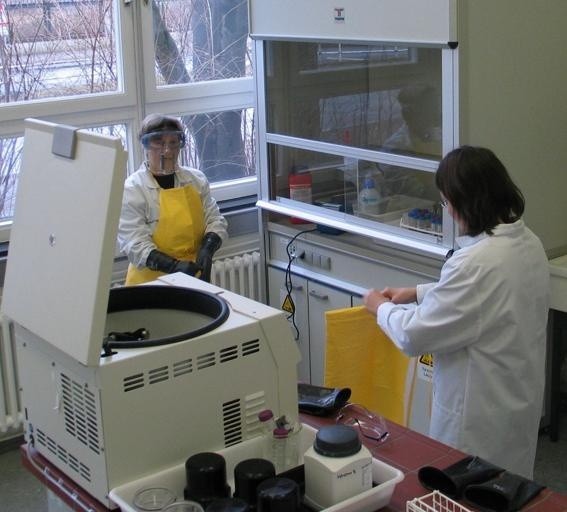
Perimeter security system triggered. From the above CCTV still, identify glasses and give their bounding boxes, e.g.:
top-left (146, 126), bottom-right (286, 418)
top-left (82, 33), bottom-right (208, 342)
top-left (440, 199), bottom-right (450, 207)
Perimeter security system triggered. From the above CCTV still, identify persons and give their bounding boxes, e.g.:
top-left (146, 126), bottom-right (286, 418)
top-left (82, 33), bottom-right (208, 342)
top-left (116, 114), bottom-right (228, 286)
top-left (366, 83), bottom-right (443, 226)
top-left (365, 145), bottom-right (549, 482)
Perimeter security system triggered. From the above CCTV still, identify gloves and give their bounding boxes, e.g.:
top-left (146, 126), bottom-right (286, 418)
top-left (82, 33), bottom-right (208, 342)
top-left (298, 383), bottom-right (351, 416)
top-left (417, 455), bottom-right (546, 510)
top-left (146, 231), bottom-right (222, 282)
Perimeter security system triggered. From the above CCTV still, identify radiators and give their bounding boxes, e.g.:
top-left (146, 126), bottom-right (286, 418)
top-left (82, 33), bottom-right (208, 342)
top-left (212, 251), bottom-right (263, 303)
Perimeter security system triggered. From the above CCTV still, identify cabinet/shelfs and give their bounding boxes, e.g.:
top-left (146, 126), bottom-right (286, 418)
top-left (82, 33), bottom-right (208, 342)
top-left (265, 265), bottom-right (352, 386)
top-left (253, 34), bottom-right (567, 258)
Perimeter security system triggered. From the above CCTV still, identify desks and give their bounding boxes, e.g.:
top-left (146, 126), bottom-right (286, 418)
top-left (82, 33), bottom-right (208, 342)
top-left (19, 380), bottom-right (567, 512)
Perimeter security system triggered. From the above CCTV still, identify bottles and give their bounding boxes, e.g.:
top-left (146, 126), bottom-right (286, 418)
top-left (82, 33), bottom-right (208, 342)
top-left (185, 409), bottom-right (372, 512)
top-left (289, 166), bottom-right (314, 225)
top-left (133, 486), bottom-right (205, 511)
top-left (402, 208), bottom-right (442, 235)
top-left (358, 178), bottom-right (380, 217)
